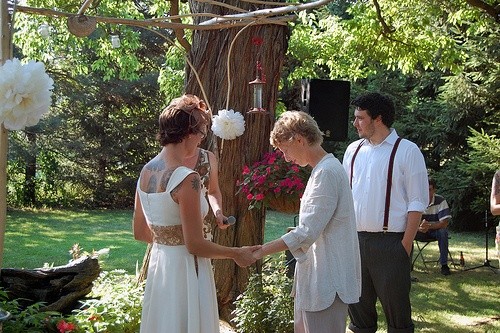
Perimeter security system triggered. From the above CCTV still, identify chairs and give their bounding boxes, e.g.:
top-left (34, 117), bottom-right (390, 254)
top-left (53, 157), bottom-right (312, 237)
top-left (411, 234), bottom-right (457, 272)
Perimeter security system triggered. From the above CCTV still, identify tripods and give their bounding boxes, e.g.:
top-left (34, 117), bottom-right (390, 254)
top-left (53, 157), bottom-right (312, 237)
top-left (462, 186), bottom-right (498, 271)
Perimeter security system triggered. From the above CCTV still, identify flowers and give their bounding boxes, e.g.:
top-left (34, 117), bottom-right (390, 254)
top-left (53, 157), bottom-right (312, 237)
top-left (210, 109), bottom-right (245, 141)
top-left (234, 149), bottom-right (313, 212)
top-left (0, 57), bottom-right (54, 130)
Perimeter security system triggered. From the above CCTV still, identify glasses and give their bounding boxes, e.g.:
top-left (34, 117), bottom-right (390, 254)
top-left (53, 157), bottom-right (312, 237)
top-left (197, 129), bottom-right (208, 139)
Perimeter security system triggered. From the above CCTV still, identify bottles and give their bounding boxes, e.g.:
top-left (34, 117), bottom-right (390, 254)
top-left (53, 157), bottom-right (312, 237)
top-left (460, 251), bottom-right (464, 268)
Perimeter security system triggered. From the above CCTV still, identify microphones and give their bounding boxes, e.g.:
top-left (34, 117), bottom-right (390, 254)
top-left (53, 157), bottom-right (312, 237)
top-left (223, 216), bottom-right (236, 226)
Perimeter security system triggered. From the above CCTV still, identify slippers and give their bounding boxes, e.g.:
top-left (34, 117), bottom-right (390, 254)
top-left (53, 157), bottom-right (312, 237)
top-left (441, 265), bottom-right (450, 276)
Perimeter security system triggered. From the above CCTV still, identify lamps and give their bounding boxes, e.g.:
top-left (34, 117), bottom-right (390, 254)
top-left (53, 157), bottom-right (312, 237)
top-left (246, 61), bottom-right (266, 113)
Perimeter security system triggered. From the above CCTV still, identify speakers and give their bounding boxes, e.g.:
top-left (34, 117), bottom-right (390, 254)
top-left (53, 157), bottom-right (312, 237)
top-left (308, 78), bottom-right (350, 141)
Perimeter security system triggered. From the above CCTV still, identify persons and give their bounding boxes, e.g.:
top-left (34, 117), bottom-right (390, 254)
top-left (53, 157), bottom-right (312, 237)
top-left (410, 179), bottom-right (451, 275)
top-left (133, 95), bottom-right (262, 333)
top-left (343, 93), bottom-right (430, 333)
top-left (490, 170), bottom-right (500, 259)
top-left (184, 148), bottom-right (230, 229)
top-left (253, 111), bottom-right (362, 333)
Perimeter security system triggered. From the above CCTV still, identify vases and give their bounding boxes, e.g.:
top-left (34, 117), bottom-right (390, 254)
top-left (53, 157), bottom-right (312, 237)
top-left (267, 195), bottom-right (299, 214)
